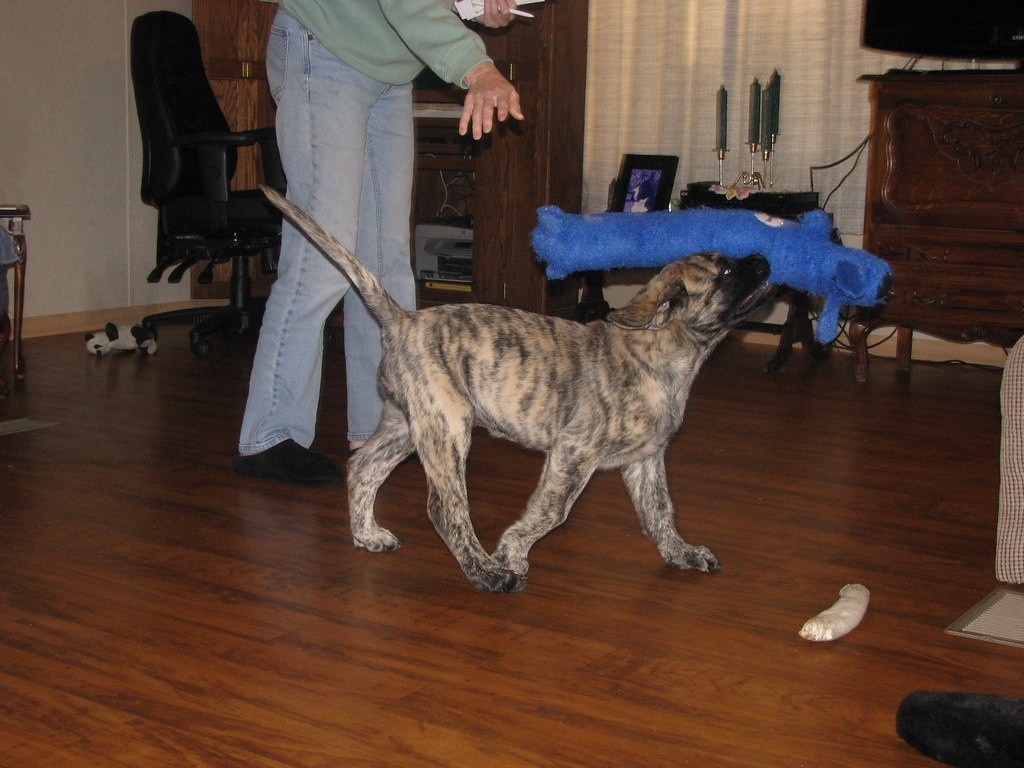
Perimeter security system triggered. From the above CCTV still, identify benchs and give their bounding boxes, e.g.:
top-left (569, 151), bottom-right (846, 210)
top-left (564, 191), bottom-right (830, 374)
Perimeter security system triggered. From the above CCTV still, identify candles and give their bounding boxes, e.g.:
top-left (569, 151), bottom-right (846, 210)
top-left (748, 76), bottom-right (761, 142)
top-left (759, 67), bottom-right (780, 149)
top-left (714, 84), bottom-right (729, 149)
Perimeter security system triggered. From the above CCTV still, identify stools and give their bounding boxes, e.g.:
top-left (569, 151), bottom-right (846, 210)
top-left (0, 204), bottom-right (31, 397)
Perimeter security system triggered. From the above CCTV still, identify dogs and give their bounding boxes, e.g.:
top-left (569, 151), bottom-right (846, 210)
top-left (251, 182), bottom-right (774, 592)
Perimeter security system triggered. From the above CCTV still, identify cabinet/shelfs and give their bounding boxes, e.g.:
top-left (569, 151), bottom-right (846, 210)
top-left (849, 68), bottom-right (1024, 383)
top-left (189, 0), bottom-right (587, 313)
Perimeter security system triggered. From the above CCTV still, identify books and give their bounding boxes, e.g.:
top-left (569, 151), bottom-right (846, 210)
top-left (413, 102), bottom-right (463, 118)
top-left (425, 282), bottom-right (472, 293)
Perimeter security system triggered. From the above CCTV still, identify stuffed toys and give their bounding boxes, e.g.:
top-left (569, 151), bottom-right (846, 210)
top-left (85, 323), bottom-right (157, 356)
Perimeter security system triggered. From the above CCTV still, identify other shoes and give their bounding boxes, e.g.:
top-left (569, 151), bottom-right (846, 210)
top-left (896, 688), bottom-right (1024, 768)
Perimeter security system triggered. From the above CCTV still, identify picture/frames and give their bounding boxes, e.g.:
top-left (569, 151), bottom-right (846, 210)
top-left (610, 153), bottom-right (680, 212)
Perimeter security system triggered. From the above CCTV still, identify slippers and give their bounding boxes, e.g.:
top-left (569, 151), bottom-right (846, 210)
top-left (236, 437), bottom-right (335, 486)
top-left (346, 445), bottom-right (419, 464)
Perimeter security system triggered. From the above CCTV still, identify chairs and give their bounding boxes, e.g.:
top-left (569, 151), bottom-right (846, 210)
top-left (131, 11), bottom-right (336, 358)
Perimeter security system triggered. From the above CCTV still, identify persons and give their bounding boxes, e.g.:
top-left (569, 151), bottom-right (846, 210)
top-left (241, 0), bottom-right (526, 487)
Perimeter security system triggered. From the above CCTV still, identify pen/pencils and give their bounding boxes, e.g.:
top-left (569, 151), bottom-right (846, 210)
top-left (471, 0), bottom-right (535, 18)
